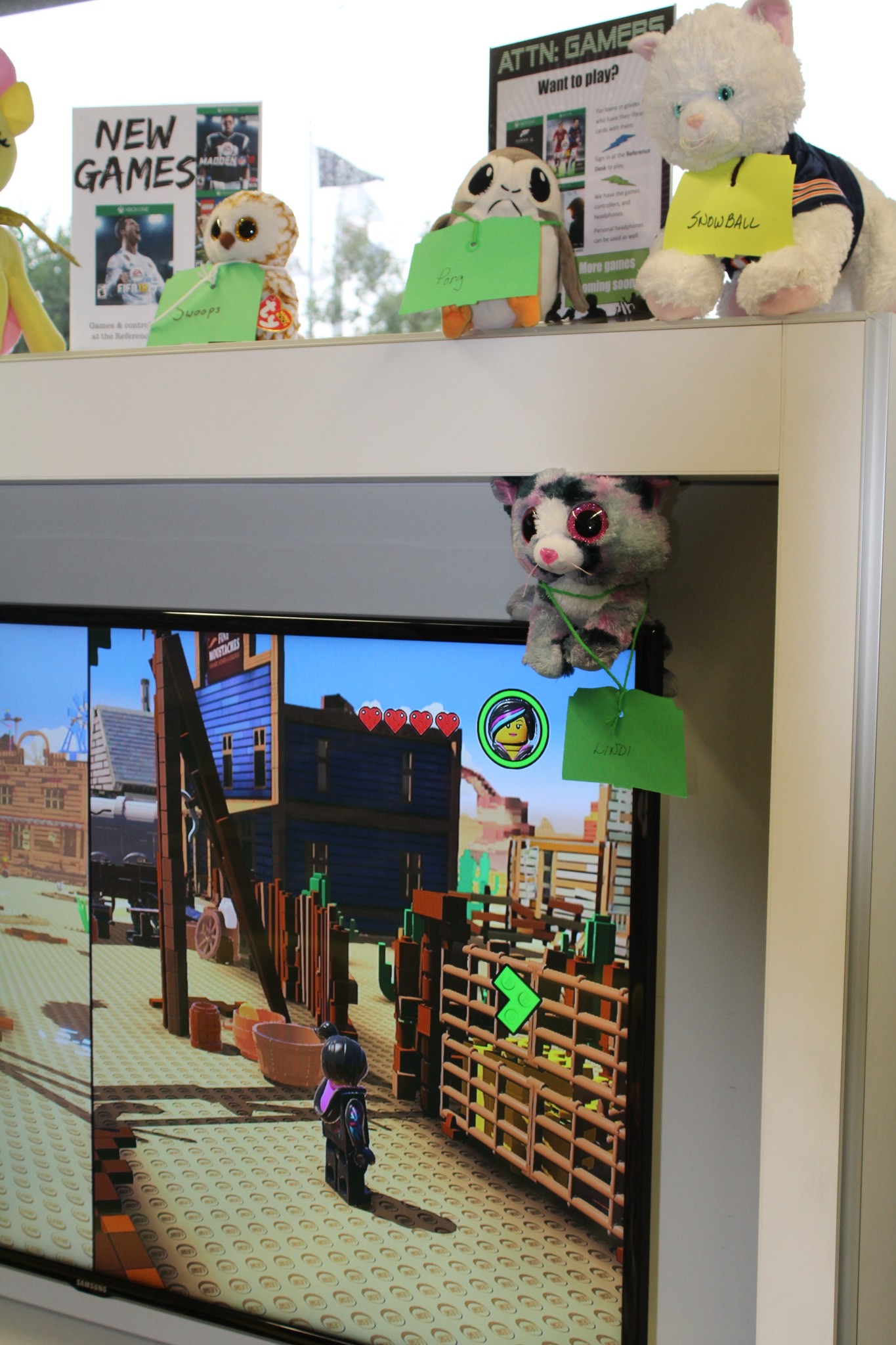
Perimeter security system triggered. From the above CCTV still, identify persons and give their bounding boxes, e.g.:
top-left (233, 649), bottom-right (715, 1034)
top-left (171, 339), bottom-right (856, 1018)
top-left (101, 217), bottom-right (164, 305)
top-left (550, 119), bottom-right (571, 170)
top-left (564, 120), bottom-right (583, 171)
top-left (198, 113), bottom-right (251, 192)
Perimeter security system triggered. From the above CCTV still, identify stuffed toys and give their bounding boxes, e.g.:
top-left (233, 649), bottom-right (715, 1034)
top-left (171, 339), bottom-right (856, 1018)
top-left (622, 1), bottom-right (896, 319)
top-left (488, 476), bottom-right (680, 678)
top-left (0, 48), bottom-right (70, 357)
top-left (426, 145), bottom-right (592, 334)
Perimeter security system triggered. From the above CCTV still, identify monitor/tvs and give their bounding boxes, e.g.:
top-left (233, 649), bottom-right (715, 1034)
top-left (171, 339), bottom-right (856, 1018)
top-left (1, 598), bottom-right (671, 1344)
